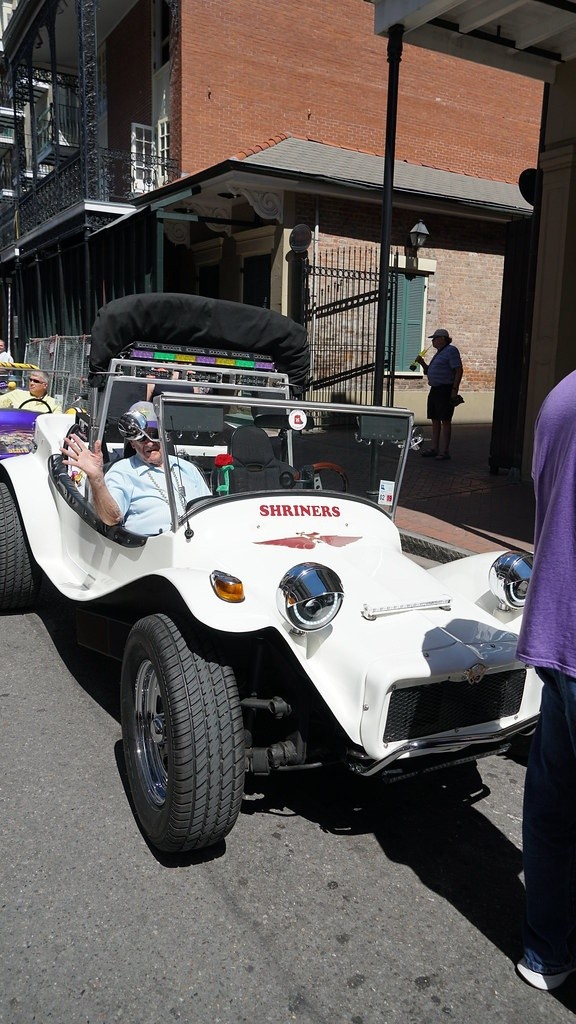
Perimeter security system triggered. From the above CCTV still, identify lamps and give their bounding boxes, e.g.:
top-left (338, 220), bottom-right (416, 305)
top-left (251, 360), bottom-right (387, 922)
top-left (409, 220), bottom-right (429, 258)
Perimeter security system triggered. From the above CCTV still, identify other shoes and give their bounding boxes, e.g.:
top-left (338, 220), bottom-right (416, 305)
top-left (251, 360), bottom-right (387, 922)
top-left (435, 454), bottom-right (451, 459)
top-left (421, 448), bottom-right (438, 456)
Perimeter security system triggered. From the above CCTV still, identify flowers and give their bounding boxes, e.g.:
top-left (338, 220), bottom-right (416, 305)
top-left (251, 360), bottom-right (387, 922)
top-left (214, 453), bottom-right (235, 496)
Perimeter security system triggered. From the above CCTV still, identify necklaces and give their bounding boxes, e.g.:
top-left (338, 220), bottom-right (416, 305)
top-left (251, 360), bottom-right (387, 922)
top-left (148, 471), bottom-right (185, 514)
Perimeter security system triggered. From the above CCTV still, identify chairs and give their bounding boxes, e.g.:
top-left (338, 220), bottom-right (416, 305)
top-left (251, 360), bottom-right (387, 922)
top-left (209, 425), bottom-right (302, 497)
top-left (104, 437), bottom-right (176, 474)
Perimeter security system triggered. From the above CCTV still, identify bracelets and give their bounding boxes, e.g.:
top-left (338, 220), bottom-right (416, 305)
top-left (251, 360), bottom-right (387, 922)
top-left (452, 387), bottom-right (458, 392)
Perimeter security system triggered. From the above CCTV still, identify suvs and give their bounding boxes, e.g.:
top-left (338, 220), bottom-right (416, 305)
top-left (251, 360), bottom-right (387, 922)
top-left (1, 290), bottom-right (546, 852)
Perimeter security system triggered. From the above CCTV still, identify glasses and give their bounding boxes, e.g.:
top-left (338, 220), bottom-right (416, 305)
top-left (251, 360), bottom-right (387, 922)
top-left (29, 378), bottom-right (43, 384)
top-left (137, 431), bottom-right (159, 442)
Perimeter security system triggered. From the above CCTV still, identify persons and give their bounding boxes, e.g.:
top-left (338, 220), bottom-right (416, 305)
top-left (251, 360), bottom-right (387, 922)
top-left (0, 340), bottom-right (14, 363)
top-left (0, 371), bottom-right (62, 415)
top-left (58, 401), bottom-right (213, 536)
top-left (417, 330), bottom-right (463, 460)
top-left (515, 371), bottom-right (576, 991)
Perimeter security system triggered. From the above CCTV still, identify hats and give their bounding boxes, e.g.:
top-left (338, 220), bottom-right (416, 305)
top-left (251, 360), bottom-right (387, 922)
top-left (128, 401), bottom-right (160, 428)
top-left (428, 329), bottom-right (452, 343)
